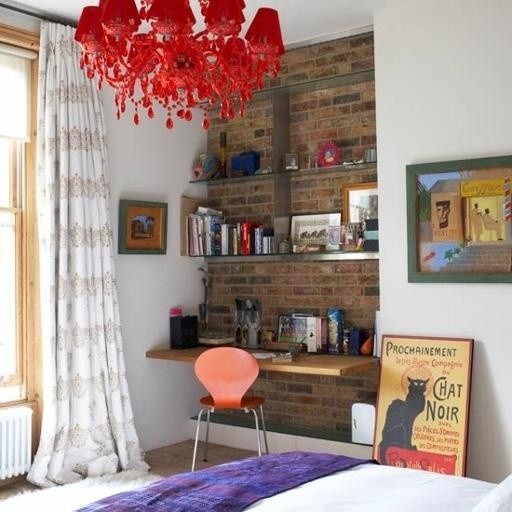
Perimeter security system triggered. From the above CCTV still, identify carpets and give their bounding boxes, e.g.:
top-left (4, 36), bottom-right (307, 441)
top-left (0, 467), bottom-right (165, 512)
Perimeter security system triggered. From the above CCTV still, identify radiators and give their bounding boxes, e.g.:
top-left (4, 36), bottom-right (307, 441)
top-left (0, 404), bottom-right (35, 481)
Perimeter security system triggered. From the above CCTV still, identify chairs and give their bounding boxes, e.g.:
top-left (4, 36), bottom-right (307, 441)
top-left (189, 347), bottom-right (267, 474)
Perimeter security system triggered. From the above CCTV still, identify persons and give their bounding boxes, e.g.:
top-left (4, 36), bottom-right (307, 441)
top-left (481, 208), bottom-right (505, 242)
top-left (470, 203), bottom-right (483, 242)
top-left (435, 200), bottom-right (450, 230)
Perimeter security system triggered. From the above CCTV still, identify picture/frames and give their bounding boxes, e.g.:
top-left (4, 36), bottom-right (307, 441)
top-left (371, 334), bottom-right (475, 478)
top-left (406, 153), bottom-right (511, 285)
top-left (118, 199), bottom-right (168, 255)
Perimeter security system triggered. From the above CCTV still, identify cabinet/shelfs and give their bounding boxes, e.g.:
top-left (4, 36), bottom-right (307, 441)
top-left (187, 69), bottom-right (379, 264)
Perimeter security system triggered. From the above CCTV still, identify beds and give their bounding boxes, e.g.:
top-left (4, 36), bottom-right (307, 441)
top-left (72, 447), bottom-right (512, 511)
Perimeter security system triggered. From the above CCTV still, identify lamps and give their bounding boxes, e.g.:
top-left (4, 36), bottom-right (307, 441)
top-left (71, 1), bottom-right (286, 132)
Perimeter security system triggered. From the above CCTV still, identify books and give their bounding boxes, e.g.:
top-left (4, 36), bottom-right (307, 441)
top-left (185, 206), bottom-right (274, 257)
